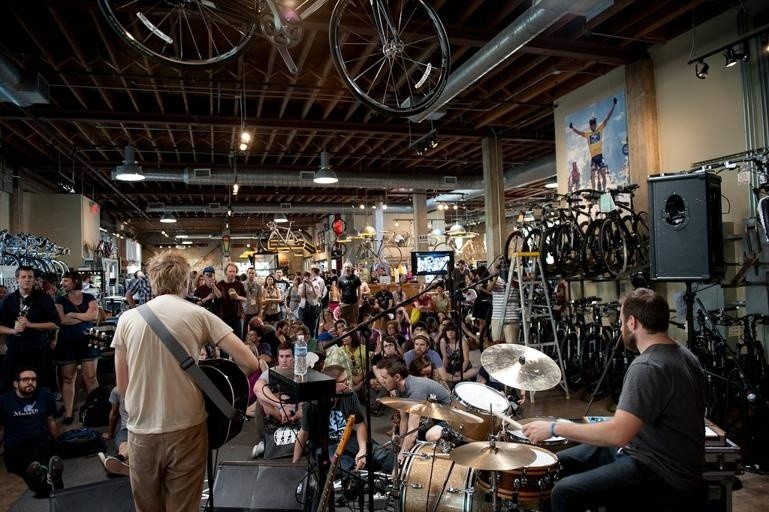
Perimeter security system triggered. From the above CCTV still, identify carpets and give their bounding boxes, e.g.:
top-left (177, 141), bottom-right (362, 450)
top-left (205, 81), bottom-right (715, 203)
top-left (7, 404), bottom-right (401, 511)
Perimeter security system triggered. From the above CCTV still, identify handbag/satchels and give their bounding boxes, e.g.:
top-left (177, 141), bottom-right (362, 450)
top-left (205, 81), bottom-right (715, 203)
top-left (301, 301), bottom-right (322, 321)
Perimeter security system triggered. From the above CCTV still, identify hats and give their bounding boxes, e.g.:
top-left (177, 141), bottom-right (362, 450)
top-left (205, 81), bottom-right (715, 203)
top-left (412, 330), bottom-right (431, 345)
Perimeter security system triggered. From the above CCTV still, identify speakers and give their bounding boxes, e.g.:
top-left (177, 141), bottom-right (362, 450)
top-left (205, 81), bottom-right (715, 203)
top-left (647, 172), bottom-right (724, 281)
top-left (207, 459), bottom-right (311, 511)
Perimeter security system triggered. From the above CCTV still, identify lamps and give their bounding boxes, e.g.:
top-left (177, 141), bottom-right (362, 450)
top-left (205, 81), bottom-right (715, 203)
top-left (273, 213), bottom-right (288, 223)
top-left (351, 188), bottom-right (387, 210)
top-left (312, 136), bottom-right (338, 184)
top-left (393, 205), bottom-right (444, 228)
top-left (408, 115), bottom-right (439, 156)
top-left (449, 201), bottom-right (466, 234)
top-left (225, 175), bottom-right (240, 230)
top-left (431, 203), bottom-right (442, 236)
top-left (159, 211), bottom-right (177, 223)
top-left (686, 0), bottom-right (769, 78)
top-left (56, 151), bottom-right (77, 193)
top-left (435, 189), bottom-right (466, 210)
top-left (239, 120), bottom-right (250, 150)
top-left (114, 129), bottom-right (146, 182)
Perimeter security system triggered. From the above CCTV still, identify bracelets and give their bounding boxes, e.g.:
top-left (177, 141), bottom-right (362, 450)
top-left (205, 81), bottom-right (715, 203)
top-left (549, 421), bottom-right (558, 439)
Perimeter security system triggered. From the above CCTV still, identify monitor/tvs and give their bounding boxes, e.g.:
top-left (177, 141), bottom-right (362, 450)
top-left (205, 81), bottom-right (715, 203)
top-left (411, 252), bottom-right (455, 274)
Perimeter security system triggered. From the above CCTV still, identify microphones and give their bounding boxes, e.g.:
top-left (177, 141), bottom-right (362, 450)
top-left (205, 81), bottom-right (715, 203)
top-left (500, 258), bottom-right (510, 279)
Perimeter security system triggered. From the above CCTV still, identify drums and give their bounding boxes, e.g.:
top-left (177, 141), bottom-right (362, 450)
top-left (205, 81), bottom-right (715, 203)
top-left (505, 416), bottom-right (578, 452)
top-left (474, 446), bottom-right (563, 502)
top-left (394, 440), bottom-right (491, 512)
top-left (446, 386), bottom-right (512, 441)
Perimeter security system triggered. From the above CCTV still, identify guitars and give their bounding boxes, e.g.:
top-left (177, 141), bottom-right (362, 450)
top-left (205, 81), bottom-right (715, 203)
top-left (83, 327), bottom-right (251, 447)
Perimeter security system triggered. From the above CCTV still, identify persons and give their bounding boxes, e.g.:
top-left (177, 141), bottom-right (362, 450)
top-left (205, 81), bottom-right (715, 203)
top-left (1, 261), bottom-right (263, 494)
top-left (569, 97), bottom-right (617, 191)
top-left (623, 136), bottom-right (630, 184)
top-left (110, 249), bottom-right (259, 511)
top-left (237, 260), bottom-right (522, 496)
top-left (568, 161), bottom-right (581, 194)
top-left (522, 286), bottom-right (710, 512)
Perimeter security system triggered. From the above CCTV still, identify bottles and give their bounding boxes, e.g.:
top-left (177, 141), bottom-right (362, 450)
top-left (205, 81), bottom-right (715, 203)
top-left (294, 334), bottom-right (308, 377)
top-left (13, 304), bottom-right (30, 334)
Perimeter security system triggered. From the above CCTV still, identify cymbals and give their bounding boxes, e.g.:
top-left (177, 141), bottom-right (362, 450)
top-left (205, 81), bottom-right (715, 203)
top-left (450, 442), bottom-right (536, 471)
top-left (480, 342), bottom-right (561, 390)
top-left (378, 396), bottom-right (484, 425)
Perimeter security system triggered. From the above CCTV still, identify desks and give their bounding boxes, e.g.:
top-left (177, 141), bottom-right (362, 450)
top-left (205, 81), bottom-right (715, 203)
top-left (366, 281), bottom-right (417, 329)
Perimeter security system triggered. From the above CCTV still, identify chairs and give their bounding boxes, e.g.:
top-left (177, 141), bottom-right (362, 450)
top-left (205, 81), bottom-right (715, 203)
top-left (363, 295), bottom-right (384, 329)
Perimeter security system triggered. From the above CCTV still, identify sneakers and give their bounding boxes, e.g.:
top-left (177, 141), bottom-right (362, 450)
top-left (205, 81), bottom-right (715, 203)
top-left (24, 460), bottom-right (48, 496)
top-left (45, 454), bottom-right (66, 493)
top-left (96, 450), bottom-right (129, 477)
top-left (61, 415), bottom-right (74, 426)
top-left (250, 440), bottom-right (266, 460)
